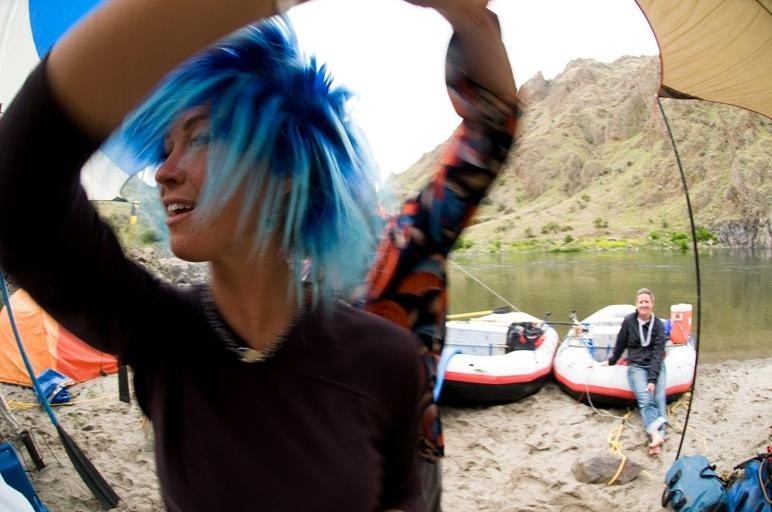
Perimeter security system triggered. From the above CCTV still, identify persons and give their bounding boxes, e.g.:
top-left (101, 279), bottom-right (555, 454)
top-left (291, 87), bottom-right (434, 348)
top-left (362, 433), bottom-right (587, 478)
top-left (599, 288), bottom-right (670, 448)
top-left (1, 0), bottom-right (522, 512)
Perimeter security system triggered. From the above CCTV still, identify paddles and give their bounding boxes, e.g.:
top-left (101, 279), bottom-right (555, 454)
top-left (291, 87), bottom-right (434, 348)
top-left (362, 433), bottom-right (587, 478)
top-left (1, 281), bottom-right (122, 507)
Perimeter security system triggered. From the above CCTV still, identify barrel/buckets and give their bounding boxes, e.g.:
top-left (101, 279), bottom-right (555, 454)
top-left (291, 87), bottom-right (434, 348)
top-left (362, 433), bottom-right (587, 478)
top-left (669, 303), bottom-right (693, 343)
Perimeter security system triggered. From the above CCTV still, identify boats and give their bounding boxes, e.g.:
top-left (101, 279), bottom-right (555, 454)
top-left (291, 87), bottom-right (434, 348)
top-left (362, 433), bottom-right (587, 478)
top-left (438, 309), bottom-right (558, 399)
top-left (553, 303), bottom-right (697, 399)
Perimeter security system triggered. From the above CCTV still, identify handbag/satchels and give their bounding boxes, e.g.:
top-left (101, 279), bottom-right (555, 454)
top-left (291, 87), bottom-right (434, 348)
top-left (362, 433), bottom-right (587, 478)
top-left (665, 455), bottom-right (730, 511)
